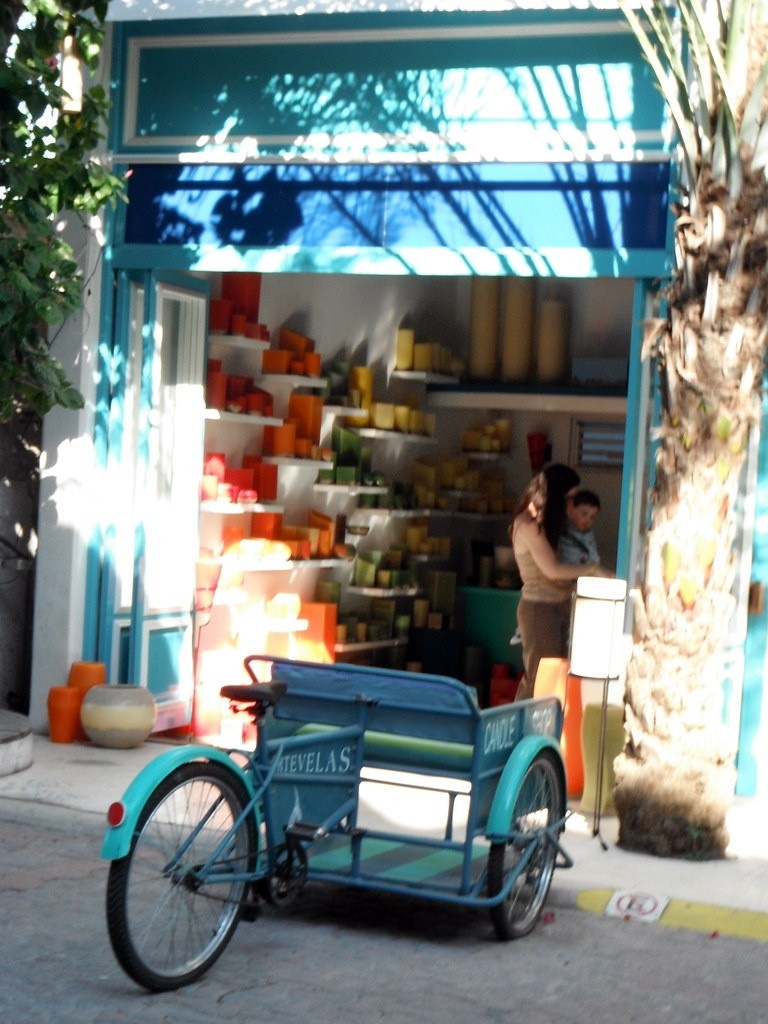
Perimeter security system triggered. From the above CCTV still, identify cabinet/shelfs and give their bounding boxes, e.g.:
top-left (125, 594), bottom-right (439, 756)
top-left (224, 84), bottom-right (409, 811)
top-left (195, 334), bottom-right (516, 738)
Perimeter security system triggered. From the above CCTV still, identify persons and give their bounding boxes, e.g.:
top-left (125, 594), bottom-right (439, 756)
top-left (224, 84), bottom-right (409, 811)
top-left (511, 464), bottom-right (616, 759)
top-left (566, 489), bottom-right (601, 567)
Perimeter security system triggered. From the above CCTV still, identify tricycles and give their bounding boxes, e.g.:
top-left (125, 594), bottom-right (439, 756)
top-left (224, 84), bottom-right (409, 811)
top-left (99, 653), bottom-right (577, 993)
